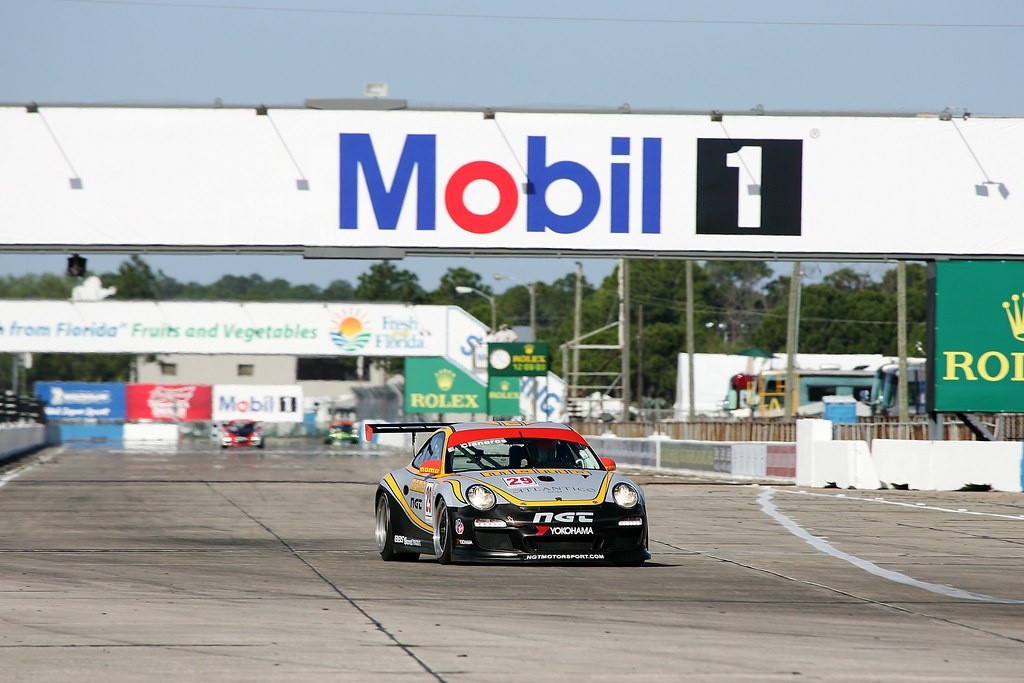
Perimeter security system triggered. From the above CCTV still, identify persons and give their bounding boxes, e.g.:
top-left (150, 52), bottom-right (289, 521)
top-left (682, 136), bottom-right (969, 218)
top-left (521, 440), bottom-right (576, 469)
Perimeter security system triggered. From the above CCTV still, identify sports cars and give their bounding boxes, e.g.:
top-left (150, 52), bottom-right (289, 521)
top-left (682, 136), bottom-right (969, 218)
top-left (323, 419), bottom-right (360, 446)
top-left (220, 419), bottom-right (265, 450)
top-left (365, 423), bottom-right (653, 567)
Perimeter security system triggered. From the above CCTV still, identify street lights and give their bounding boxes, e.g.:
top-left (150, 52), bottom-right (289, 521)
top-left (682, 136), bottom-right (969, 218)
top-left (495, 272), bottom-right (536, 342)
top-left (455, 287), bottom-right (496, 335)
